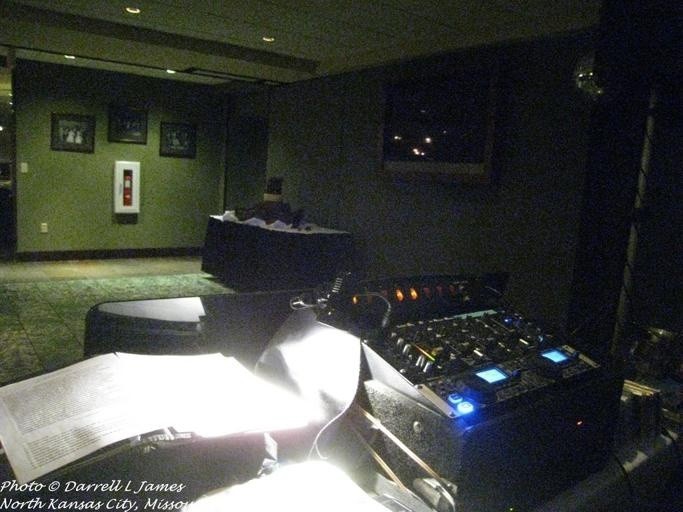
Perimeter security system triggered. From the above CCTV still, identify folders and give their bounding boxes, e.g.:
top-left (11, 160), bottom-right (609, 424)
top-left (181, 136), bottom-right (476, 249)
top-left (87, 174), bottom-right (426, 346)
top-left (1, 350), bottom-right (331, 487)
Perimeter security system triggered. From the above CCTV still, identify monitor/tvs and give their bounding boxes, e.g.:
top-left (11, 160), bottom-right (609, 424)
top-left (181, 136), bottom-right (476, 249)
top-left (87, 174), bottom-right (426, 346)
top-left (383, 89), bottom-right (489, 165)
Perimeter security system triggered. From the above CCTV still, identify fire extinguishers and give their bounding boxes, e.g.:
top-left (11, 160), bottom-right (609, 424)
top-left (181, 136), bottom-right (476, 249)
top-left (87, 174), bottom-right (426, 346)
top-left (124, 171), bottom-right (132, 206)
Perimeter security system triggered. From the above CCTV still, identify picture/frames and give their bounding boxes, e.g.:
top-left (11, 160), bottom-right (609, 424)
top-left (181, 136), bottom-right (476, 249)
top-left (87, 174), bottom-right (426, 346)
top-left (108, 100), bottom-right (151, 143)
top-left (158, 122), bottom-right (197, 158)
top-left (52, 111), bottom-right (96, 153)
